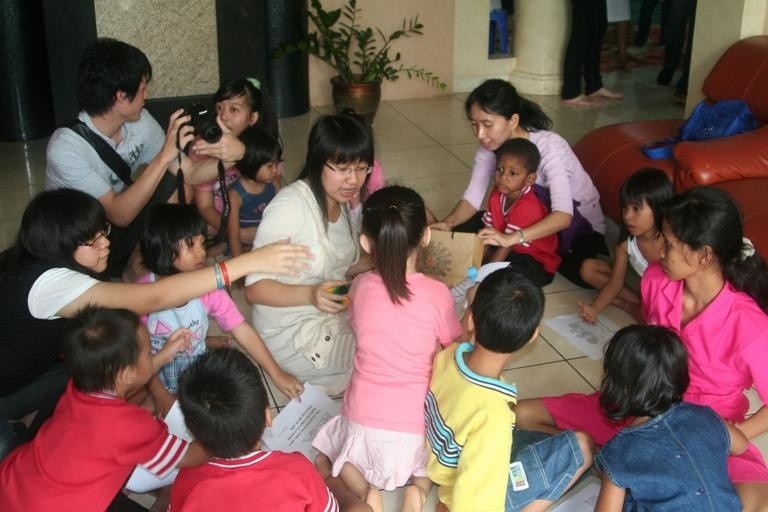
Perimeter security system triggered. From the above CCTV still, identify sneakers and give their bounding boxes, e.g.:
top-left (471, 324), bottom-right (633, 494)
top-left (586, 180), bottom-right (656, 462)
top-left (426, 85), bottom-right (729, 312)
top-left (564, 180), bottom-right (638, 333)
top-left (656, 63), bottom-right (688, 104)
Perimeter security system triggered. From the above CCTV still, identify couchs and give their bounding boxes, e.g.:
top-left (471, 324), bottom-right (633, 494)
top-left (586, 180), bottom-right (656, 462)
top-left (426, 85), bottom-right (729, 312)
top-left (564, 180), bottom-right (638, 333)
top-left (671, 126), bottom-right (768, 261)
top-left (571, 31), bottom-right (766, 227)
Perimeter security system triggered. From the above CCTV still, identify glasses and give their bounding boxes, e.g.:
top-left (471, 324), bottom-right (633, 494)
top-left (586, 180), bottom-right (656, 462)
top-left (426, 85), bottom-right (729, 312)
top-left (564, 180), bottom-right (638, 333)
top-left (324, 163), bottom-right (374, 176)
top-left (77, 221), bottom-right (112, 249)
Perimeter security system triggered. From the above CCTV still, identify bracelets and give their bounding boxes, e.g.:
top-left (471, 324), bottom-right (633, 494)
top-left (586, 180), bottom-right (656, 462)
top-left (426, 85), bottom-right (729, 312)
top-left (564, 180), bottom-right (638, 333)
top-left (220, 262), bottom-right (232, 286)
top-left (443, 217), bottom-right (454, 228)
top-left (214, 261), bottom-right (223, 290)
top-left (517, 230), bottom-right (524, 246)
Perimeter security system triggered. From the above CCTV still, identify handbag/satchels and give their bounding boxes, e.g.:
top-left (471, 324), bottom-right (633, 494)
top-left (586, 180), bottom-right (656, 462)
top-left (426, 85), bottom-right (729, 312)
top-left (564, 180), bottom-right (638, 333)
top-left (678, 99), bottom-right (758, 141)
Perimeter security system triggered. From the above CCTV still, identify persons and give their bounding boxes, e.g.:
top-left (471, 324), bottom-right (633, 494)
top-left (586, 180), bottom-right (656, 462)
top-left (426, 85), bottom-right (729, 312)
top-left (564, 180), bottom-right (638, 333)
top-left (578, 165), bottom-right (679, 325)
top-left (228, 126), bottom-right (282, 288)
top-left (310, 186), bottom-right (482, 511)
top-left (511, 184), bottom-right (768, 512)
top-left (479, 137), bottom-right (561, 287)
top-left (165, 346), bottom-right (368, 511)
top-left (188, 76), bottom-right (284, 235)
top-left (424, 265), bottom-right (593, 511)
top-left (122, 200), bottom-right (306, 420)
top-left (594, 325), bottom-right (750, 512)
top-left (343, 107), bottom-right (384, 203)
top-left (1, 302), bottom-right (212, 511)
top-left (420, 78), bottom-right (605, 289)
top-left (560, 1), bottom-right (698, 109)
top-left (243, 115), bottom-right (370, 405)
top-left (1, 186), bottom-right (318, 433)
top-left (43, 36), bottom-right (245, 262)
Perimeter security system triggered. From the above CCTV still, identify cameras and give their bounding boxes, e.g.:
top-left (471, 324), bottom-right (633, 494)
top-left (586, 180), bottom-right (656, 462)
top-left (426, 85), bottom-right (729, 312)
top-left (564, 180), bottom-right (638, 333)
top-left (180, 101), bottom-right (223, 143)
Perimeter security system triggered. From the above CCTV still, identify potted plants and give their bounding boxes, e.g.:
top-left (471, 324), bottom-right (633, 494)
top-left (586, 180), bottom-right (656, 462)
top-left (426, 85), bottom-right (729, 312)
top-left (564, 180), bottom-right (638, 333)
top-left (271, 0), bottom-right (447, 127)
